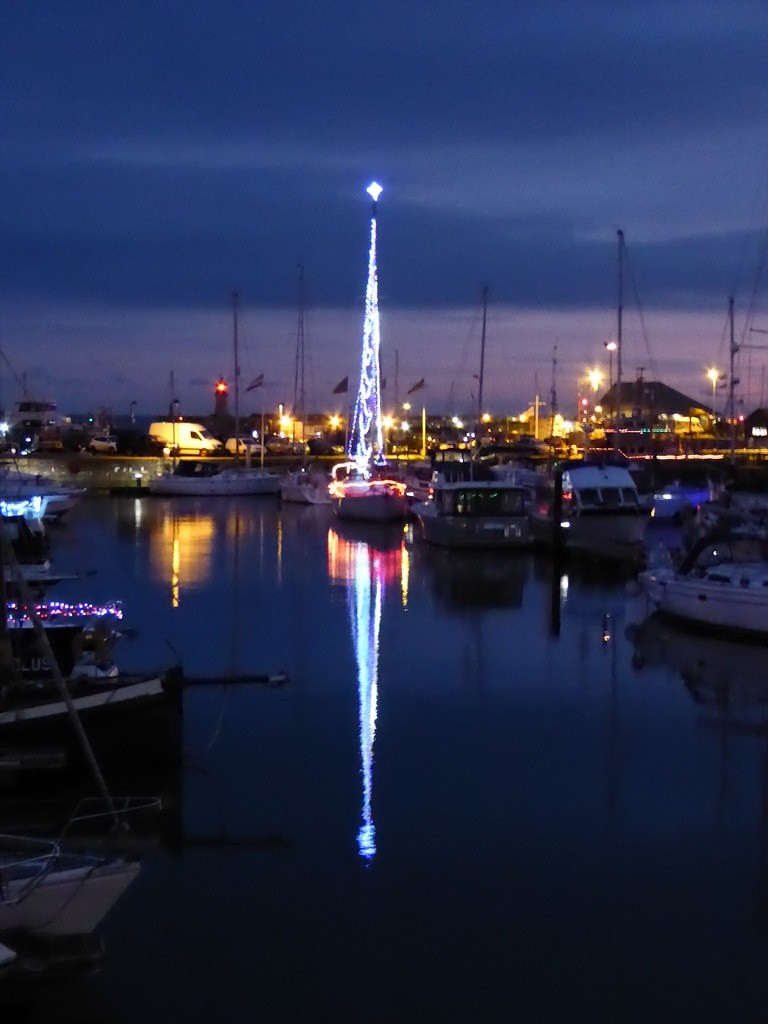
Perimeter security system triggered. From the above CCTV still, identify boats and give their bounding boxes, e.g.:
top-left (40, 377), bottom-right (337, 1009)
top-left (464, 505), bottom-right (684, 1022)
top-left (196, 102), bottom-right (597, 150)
top-left (0, 230), bottom-right (768, 958)
top-left (627, 611), bottom-right (768, 703)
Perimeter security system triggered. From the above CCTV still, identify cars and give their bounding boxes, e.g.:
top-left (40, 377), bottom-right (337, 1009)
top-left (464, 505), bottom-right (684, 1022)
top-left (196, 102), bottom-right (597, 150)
top-left (226, 437), bottom-right (268, 456)
top-left (90, 435), bottom-right (119, 455)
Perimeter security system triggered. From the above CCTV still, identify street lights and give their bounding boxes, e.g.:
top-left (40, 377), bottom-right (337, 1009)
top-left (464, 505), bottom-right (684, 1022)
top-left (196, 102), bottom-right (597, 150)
top-left (707, 368), bottom-right (721, 417)
top-left (603, 340), bottom-right (616, 429)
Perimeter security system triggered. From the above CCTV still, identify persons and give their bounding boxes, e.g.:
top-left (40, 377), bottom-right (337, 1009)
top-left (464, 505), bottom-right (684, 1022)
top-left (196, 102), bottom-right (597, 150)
top-left (53, 627), bottom-right (88, 675)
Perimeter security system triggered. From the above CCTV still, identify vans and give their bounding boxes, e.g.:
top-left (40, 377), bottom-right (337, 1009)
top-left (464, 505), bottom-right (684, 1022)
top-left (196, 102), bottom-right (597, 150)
top-left (148, 422), bottom-right (226, 457)
